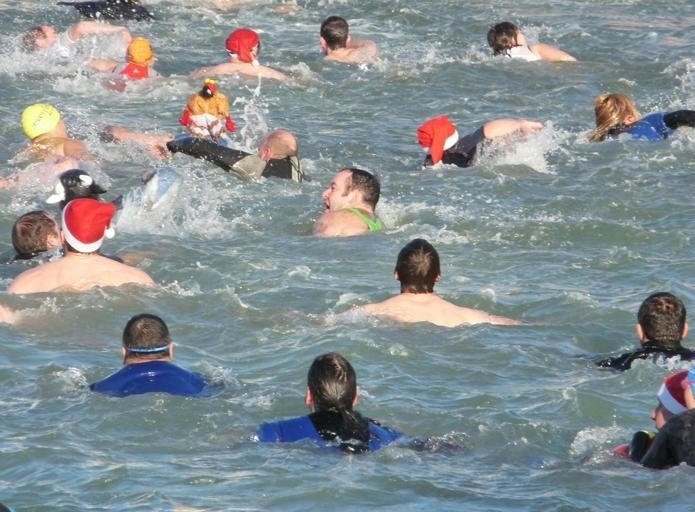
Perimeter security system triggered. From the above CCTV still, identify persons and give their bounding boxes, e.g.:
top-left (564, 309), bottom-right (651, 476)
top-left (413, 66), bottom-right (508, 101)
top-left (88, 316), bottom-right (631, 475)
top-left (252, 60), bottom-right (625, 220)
top-left (417, 117), bottom-right (544, 167)
top-left (487, 21), bottom-right (577, 61)
top-left (0, 0), bottom-right (307, 291)
top-left (254, 353), bottom-right (466, 456)
top-left (577, 292), bottom-right (695, 374)
top-left (318, 14), bottom-right (376, 61)
top-left (610, 365), bottom-right (695, 470)
top-left (89, 313), bottom-right (226, 400)
top-left (583, 92), bottom-right (695, 144)
top-left (317, 166), bottom-right (382, 235)
top-left (360, 237), bottom-right (526, 325)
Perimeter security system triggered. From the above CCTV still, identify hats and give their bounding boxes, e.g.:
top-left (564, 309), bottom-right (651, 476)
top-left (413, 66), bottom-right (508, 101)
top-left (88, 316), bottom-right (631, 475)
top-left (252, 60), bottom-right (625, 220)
top-left (656, 371), bottom-right (695, 419)
top-left (224, 28), bottom-right (261, 63)
top-left (20, 101), bottom-right (61, 140)
top-left (125, 36), bottom-right (154, 67)
top-left (45, 169), bottom-right (107, 206)
top-left (418, 116), bottom-right (460, 171)
top-left (61, 196), bottom-right (117, 253)
